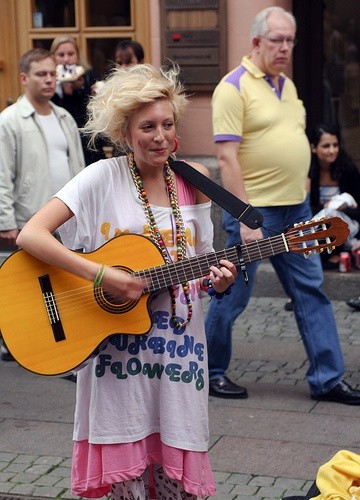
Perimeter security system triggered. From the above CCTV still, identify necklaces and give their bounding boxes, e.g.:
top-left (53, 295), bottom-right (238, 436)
top-left (128, 152), bottom-right (192, 328)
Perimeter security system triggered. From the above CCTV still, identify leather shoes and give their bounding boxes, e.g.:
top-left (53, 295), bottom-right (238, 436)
top-left (311, 381), bottom-right (360, 404)
top-left (209, 376), bottom-right (248, 398)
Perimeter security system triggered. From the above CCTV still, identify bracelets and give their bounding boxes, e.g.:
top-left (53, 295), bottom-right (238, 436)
top-left (94, 264), bottom-right (103, 284)
top-left (97, 264), bottom-right (106, 287)
top-left (209, 280), bottom-right (234, 300)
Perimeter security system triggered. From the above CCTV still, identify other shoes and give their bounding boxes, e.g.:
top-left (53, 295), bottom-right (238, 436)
top-left (347, 299), bottom-right (360, 310)
top-left (285, 298), bottom-right (293, 310)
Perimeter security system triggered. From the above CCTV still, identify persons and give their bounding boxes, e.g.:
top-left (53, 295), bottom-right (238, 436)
top-left (204, 6), bottom-right (360, 405)
top-left (308, 125), bottom-right (360, 269)
top-left (15, 62), bottom-right (238, 500)
top-left (50, 37), bottom-right (106, 167)
top-left (0, 48), bottom-right (86, 360)
top-left (115, 41), bottom-right (144, 70)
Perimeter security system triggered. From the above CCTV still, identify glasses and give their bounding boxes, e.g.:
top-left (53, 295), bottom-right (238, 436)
top-left (258, 34), bottom-right (299, 48)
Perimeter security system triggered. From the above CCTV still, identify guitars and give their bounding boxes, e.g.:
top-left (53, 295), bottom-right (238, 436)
top-left (0, 217), bottom-right (350, 375)
